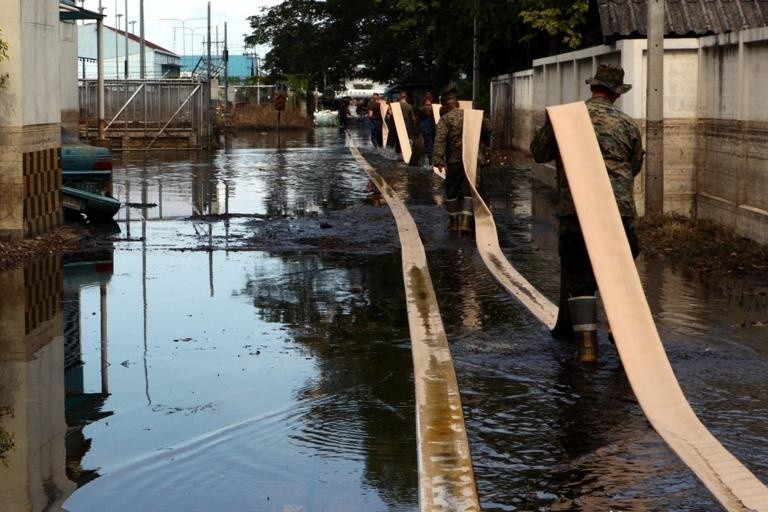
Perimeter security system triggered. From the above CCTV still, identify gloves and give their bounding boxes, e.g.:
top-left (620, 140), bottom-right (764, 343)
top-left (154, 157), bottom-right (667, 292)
top-left (434, 159), bottom-right (446, 172)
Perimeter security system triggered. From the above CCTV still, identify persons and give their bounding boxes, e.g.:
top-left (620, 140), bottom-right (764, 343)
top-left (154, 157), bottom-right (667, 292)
top-left (530, 64), bottom-right (644, 364)
top-left (340, 98), bottom-right (351, 132)
top-left (354, 86), bottom-right (478, 235)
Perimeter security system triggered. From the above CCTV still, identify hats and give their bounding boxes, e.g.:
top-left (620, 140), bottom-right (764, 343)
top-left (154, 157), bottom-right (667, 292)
top-left (441, 91), bottom-right (457, 105)
top-left (585, 62), bottom-right (632, 95)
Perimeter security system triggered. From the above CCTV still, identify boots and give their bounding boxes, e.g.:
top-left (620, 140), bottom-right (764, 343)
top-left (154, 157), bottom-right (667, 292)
top-left (458, 196), bottom-right (473, 233)
top-left (444, 198), bottom-right (460, 233)
top-left (566, 295), bottom-right (600, 364)
